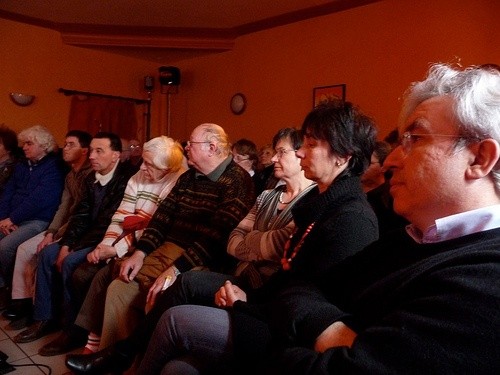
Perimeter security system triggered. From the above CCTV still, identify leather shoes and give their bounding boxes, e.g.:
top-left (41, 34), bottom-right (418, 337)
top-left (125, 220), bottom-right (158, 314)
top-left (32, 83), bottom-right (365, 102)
top-left (14, 319), bottom-right (49, 343)
top-left (2, 310), bottom-right (28, 320)
top-left (39, 327), bottom-right (84, 356)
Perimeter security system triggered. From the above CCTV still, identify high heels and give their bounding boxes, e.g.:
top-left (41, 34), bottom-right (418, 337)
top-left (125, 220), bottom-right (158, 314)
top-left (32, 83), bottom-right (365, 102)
top-left (65, 341), bottom-right (136, 375)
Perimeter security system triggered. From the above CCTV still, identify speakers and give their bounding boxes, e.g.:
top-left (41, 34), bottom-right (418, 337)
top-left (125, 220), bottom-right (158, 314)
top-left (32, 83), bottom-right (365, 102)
top-left (160, 65), bottom-right (177, 93)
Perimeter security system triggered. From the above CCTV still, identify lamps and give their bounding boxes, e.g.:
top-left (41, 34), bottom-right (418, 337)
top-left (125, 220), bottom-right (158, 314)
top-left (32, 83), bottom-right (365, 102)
top-left (11, 93), bottom-right (35, 106)
top-left (230, 93), bottom-right (247, 115)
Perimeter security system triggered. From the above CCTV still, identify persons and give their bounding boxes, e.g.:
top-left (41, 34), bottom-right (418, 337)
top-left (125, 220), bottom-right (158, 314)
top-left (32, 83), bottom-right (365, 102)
top-left (1, 98), bottom-right (500, 373)
top-left (258, 61), bottom-right (497, 374)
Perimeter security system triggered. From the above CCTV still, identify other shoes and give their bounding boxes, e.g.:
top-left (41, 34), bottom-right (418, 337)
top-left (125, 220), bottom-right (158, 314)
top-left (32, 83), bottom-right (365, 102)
top-left (9, 318), bottom-right (28, 330)
top-left (77, 335), bottom-right (101, 354)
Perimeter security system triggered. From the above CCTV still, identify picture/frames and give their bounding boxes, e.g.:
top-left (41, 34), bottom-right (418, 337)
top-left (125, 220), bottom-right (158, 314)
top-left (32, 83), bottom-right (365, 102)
top-left (313, 84), bottom-right (346, 108)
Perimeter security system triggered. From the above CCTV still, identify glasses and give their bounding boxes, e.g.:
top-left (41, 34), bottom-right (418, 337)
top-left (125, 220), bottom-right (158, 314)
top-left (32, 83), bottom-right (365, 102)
top-left (401, 131), bottom-right (483, 148)
top-left (129, 145), bottom-right (141, 151)
top-left (187, 141), bottom-right (211, 146)
top-left (274, 149), bottom-right (298, 155)
